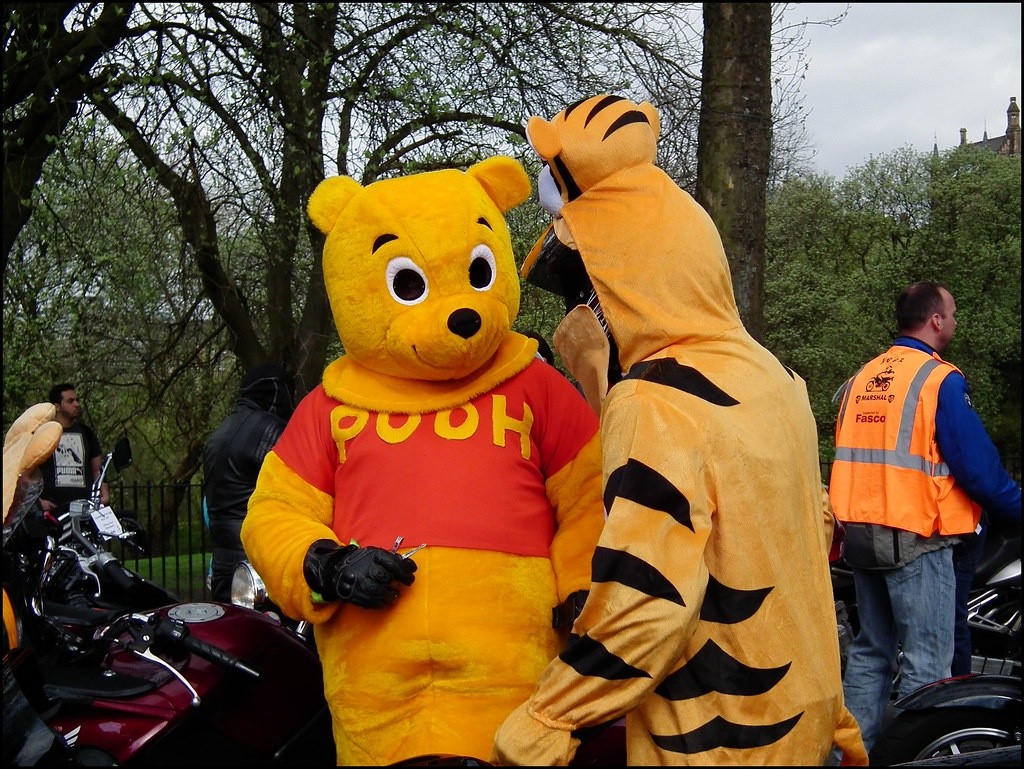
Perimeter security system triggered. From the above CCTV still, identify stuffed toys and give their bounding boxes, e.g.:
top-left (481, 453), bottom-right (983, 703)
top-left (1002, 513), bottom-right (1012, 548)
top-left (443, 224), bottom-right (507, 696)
top-left (3, 403), bottom-right (63, 650)
top-left (490, 96), bottom-right (868, 767)
top-left (239, 157), bottom-right (605, 767)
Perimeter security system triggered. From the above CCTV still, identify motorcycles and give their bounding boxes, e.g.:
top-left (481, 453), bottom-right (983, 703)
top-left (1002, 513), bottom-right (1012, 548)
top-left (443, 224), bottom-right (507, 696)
top-left (1, 432), bottom-right (338, 769)
top-left (826, 495), bottom-right (1024, 769)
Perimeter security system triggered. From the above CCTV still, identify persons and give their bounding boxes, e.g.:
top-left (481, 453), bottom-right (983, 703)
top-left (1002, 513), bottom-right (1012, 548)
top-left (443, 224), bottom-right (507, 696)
top-left (35, 383), bottom-right (110, 558)
top-left (827, 280), bottom-right (1021, 764)
top-left (204, 362), bottom-right (295, 605)
